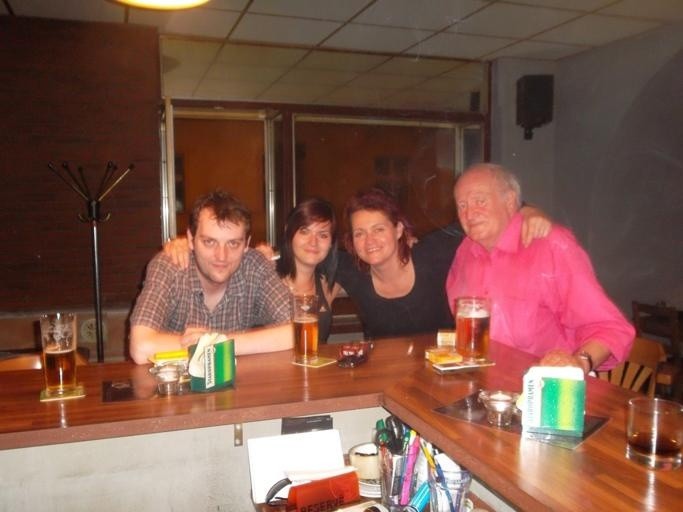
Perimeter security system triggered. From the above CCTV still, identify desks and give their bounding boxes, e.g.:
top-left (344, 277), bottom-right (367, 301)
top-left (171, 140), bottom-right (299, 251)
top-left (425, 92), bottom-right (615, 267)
top-left (646, 362), bottom-right (681, 402)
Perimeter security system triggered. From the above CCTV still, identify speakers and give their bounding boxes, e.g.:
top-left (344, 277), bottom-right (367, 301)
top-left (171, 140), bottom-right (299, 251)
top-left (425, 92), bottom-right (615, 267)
top-left (517, 75), bottom-right (553, 140)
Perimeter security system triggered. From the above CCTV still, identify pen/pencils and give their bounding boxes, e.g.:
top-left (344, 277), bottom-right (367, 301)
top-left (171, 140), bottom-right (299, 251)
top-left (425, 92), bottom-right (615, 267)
top-left (376, 416), bottom-right (419, 507)
top-left (433, 457), bottom-right (454, 512)
top-left (407, 341), bottom-right (414, 357)
top-left (419, 438), bottom-right (436, 468)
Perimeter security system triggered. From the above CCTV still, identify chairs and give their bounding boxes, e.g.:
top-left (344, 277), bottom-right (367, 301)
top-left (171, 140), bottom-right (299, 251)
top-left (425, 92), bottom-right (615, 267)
top-left (592, 337), bottom-right (666, 398)
top-left (324, 295), bottom-right (363, 335)
top-left (0, 349), bottom-right (88, 371)
top-left (626, 297), bottom-right (681, 362)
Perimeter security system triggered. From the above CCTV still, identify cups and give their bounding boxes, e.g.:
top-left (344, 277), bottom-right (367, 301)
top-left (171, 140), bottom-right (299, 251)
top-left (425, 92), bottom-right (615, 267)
top-left (626, 395), bottom-right (683, 471)
top-left (426, 463), bottom-right (471, 511)
top-left (38, 313), bottom-right (77, 394)
top-left (455, 297), bottom-right (490, 360)
top-left (336, 341), bottom-right (371, 366)
top-left (150, 361), bottom-right (186, 394)
top-left (479, 390), bottom-right (520, 425)
top-left (378, 449), bottom-right (425, 512)
top-left (435, 330), bottom-right (456, 353)
top-left (288, 294), bottom-right (322, 363)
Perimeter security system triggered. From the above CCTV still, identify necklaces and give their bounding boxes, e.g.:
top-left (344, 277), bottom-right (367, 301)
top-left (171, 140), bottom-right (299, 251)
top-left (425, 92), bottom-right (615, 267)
top-left (285, 274), bottom-right (316, 311)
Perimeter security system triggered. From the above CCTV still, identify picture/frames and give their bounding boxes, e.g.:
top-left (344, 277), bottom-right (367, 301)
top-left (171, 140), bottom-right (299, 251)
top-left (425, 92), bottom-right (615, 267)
top-left (169, 149), bottom-right (190, 217)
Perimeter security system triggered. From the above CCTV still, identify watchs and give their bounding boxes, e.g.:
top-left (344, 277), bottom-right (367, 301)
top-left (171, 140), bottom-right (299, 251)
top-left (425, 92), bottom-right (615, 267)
top-left (575, 350), bottom-right (592, 371)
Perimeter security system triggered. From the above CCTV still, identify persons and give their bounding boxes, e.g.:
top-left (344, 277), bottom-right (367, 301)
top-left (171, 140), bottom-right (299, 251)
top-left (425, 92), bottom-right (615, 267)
top-left (129, 189), bottom-right (295, 366)
top-left (256, 187), bottom-right (551, 341)
top-left (446, 164), bottom-right (636, 378)
top-left (163, 197), bottom-right (421, 345)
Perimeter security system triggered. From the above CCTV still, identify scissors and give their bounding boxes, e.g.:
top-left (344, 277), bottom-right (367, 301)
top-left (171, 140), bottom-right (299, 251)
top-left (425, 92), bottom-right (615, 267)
top-left (374, 416), bottom-right (404, 505)
